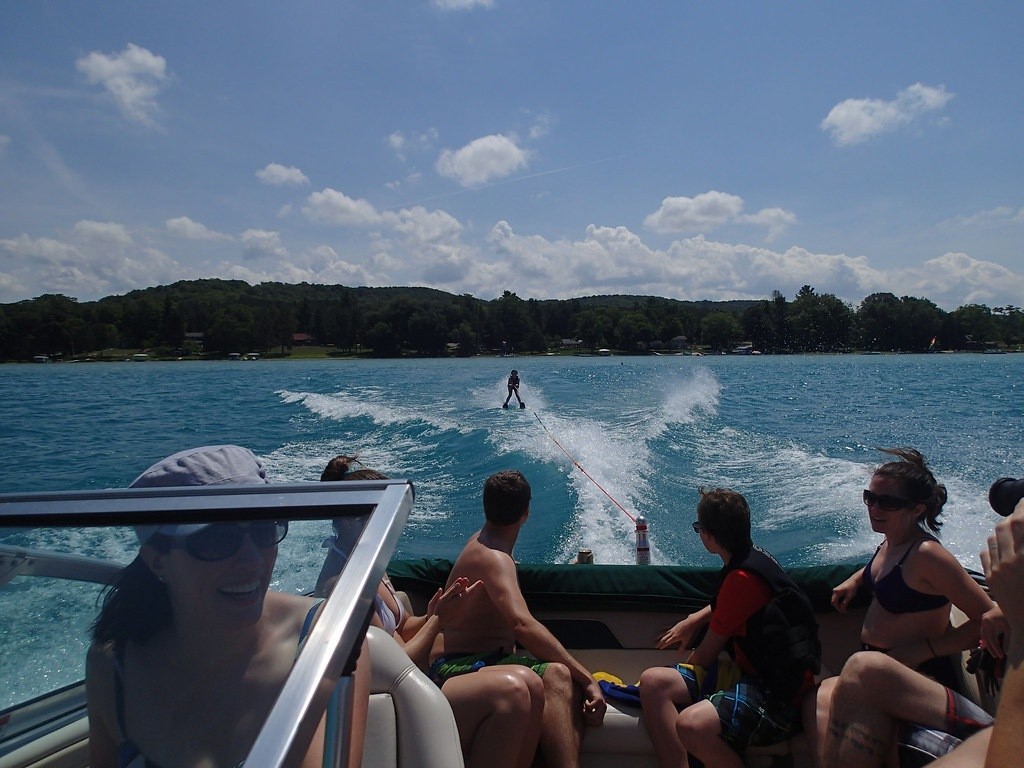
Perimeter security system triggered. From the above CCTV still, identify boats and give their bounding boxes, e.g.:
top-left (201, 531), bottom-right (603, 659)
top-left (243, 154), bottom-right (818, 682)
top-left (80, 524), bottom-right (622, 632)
top-left (1, 479), bottom-right (1004, 768)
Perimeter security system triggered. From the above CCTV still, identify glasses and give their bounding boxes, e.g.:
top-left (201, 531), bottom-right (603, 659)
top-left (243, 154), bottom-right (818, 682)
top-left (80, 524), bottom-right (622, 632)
top-left (863, 489), bottom-right (922, 512)
top-left (167, 518), bottom-right (289, 560)
top-left (692, 521), bottom-right (718, 545)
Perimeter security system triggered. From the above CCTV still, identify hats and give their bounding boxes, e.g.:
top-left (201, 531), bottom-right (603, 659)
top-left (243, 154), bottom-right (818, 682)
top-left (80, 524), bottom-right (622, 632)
top-left (123, 445), bottom-right (271, 547)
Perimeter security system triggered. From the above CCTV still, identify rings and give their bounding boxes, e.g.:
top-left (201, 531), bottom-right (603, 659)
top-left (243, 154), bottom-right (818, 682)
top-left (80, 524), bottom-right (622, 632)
top-left (841, 601), bottom-right (847, 606)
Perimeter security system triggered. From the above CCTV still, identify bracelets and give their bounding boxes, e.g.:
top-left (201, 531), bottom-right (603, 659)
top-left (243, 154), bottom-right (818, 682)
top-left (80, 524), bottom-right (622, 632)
top-left (926, 639), bottom-right (937, 658)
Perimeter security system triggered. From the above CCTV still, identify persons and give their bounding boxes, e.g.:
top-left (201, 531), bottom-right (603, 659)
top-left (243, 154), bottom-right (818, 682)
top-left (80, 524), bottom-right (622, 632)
top-left (427, 471), bottom-right (608, 768)
top-left (812, 446), bottom-right (996, 757)
top-left (83, 445), bottom-right (372, 768)
top-left (639, 488), bottom-right (822, 768)
top-left (312, 455), bottom-right (484, 665)
top-left (503, 370), bottom-right (521, 405)
top-left (822, 497), bottom-right (1024, 768)
